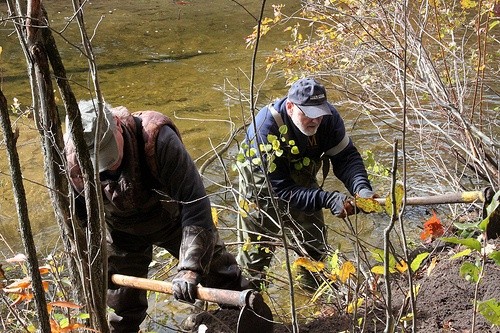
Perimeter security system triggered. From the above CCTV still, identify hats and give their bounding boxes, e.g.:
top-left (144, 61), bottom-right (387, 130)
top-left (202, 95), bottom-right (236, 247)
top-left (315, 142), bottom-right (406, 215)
top-left (66, 99), bottom-right (120, 173)
top-left (286, 77), bottom-right (335, 119)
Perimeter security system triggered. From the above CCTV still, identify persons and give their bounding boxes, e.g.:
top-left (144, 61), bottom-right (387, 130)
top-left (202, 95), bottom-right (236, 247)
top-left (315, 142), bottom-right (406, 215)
top-left (230, 78), bottom-right (378, 290)
top-left (57, 99), bottom-right (253, 333)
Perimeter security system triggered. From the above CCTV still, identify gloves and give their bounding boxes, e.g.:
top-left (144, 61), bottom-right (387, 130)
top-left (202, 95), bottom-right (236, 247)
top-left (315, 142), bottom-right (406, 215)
top-left (329, 191), bottom-right (360, 218)
top-left (106, 263), bottom-right (124, 290)
top-left (359, 189), bottom-right (381, 215)
top-left (171, 270), bottom-right (202, 305)
